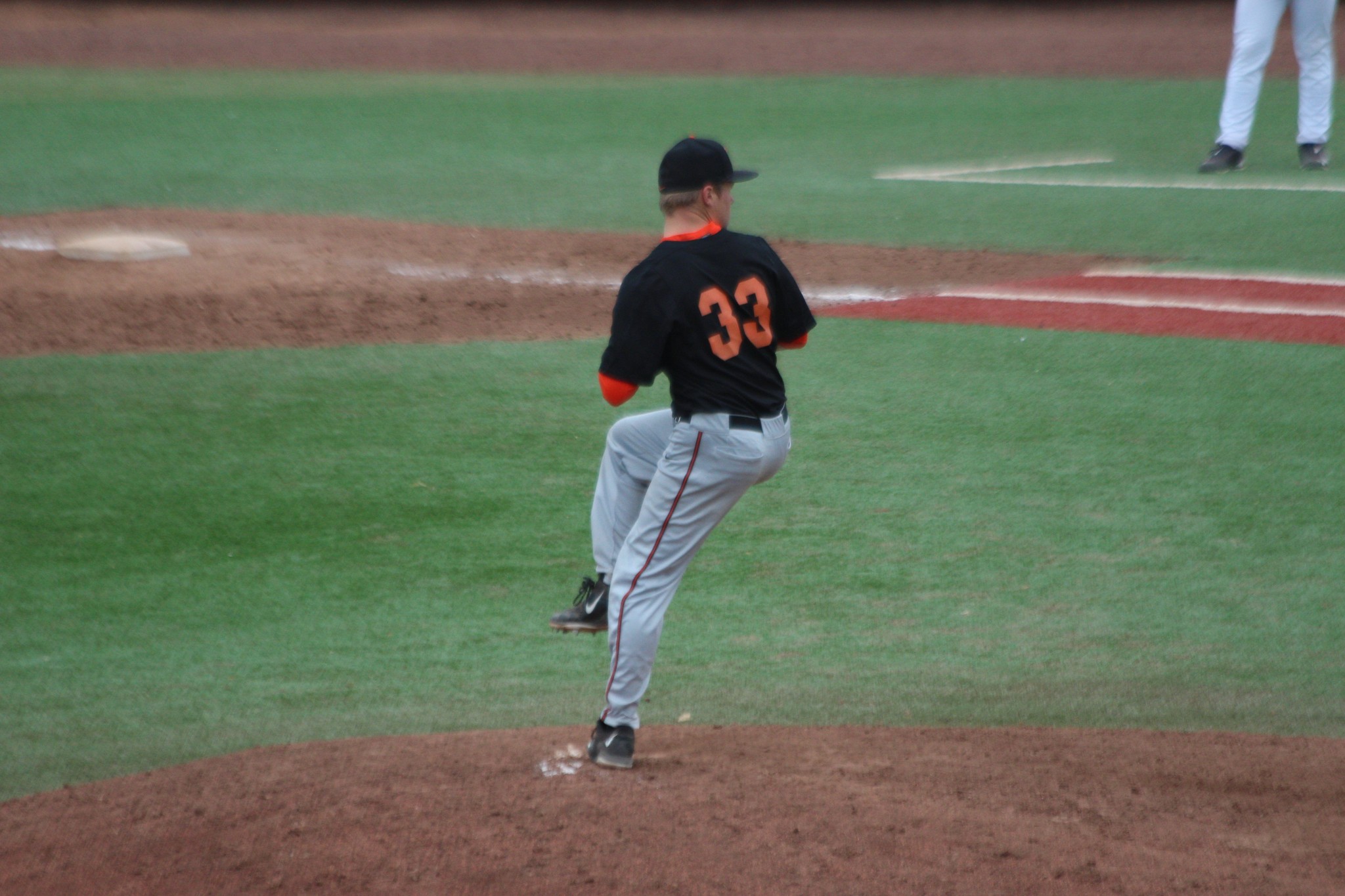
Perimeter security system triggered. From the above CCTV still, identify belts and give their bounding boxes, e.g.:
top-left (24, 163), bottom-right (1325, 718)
top-left (674, 405), bottom-right (789, 432)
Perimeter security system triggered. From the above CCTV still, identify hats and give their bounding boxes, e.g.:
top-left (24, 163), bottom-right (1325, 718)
top-left (659, 137), bottom-right (760, 194)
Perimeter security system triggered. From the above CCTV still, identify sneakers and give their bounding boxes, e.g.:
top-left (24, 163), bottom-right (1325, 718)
top-left (1298, 142), bottom-right (1327, 168)
top-left (586, 720), bottom-right (636, 769)
top-left (549, 570), bottom-right (617, 631)
top-left (1198, 143), bottom-right (1247, 175)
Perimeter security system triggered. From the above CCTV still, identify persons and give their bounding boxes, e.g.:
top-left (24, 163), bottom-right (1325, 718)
top-left (1198, 0), bottom-right (1338, 173)
top-left (551, 135), bottom-right (819, 769)
